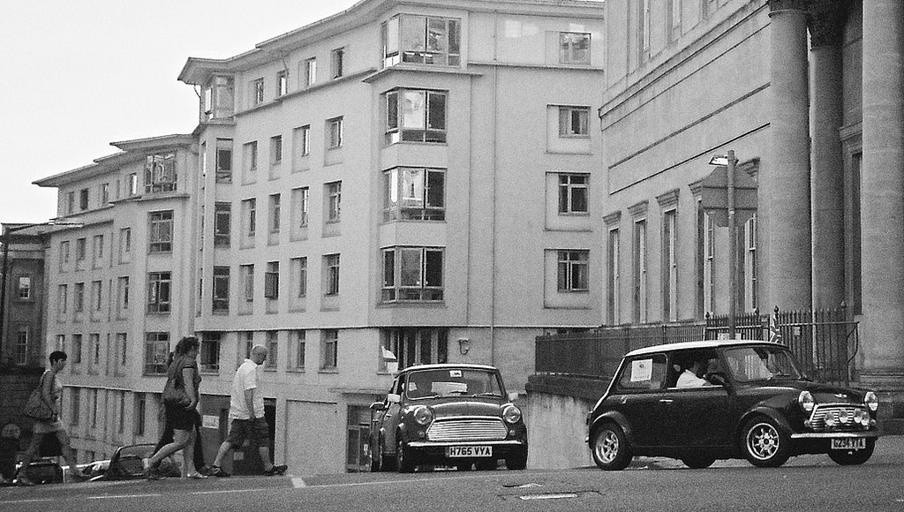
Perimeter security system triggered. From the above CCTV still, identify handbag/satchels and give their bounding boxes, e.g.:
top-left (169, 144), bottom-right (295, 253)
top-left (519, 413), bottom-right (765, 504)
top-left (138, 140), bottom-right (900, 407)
top-left (24, 389), bottom-right (56, 419)
top-left (162, 379), bottom-right (192, 407)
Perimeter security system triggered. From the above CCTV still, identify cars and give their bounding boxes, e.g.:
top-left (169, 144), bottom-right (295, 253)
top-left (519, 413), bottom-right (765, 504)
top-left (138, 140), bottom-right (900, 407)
top-left (584, 339), bottom-right (881, 471)
top-left (369, 363), bottom-right (528, 473)
top-left (12, 443), bottom-right (182, 488)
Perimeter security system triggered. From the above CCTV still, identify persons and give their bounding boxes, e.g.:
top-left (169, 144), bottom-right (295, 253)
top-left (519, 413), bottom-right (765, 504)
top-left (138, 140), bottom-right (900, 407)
top-left (143, 337), bottom-right (209, 478)
top-left (675, 350), bottom-right (712, 387)
top-left (16, 351), bottom-right (91, 485)
top-left (407, 375), bottom-right (435, 398)
top-left (147, 339), bottom-right (221, 476)
top-left (206, 346), bottom-right (288, 477)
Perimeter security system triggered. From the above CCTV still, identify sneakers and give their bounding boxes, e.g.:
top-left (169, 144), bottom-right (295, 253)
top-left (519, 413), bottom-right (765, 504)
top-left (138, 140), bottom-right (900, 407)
top-left (16, 474), bottom-right (33, 486)
top-left (208, 464), bottom-right (229, 477)
top-left (266, 464), bottom-right (288, 476)
top-left (142, 458), bottom-right (160, 481)
top-left (185, 470), bottom-right (206, 479)
top-left (72, 471), bottom-right (91, 483)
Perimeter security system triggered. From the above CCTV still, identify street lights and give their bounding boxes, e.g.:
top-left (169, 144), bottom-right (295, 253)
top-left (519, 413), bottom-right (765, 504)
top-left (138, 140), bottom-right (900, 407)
top-left (707, 149), bottom-right (739, 338)
